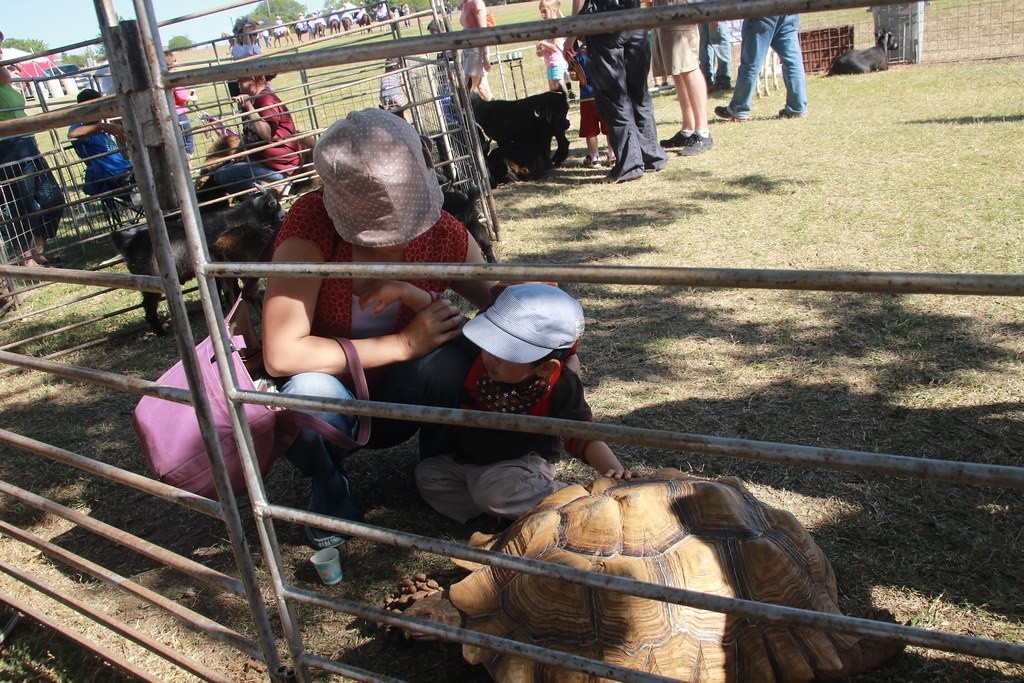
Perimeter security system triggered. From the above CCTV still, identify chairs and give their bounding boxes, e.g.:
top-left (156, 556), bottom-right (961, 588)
top-left (70, 137), bottom-right (149, 231)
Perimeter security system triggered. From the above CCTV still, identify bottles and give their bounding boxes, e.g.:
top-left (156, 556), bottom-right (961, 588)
top-left (130, 187), bottom-right (142, 205)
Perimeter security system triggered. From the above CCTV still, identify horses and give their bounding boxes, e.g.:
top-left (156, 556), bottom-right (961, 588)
top-left (221, 7), bottom-right (402, 53)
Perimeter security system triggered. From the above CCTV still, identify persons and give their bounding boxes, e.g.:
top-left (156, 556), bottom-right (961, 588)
top-left (214, 75), bottom-right (303, 204)
top-left (536, 0), bottom-right (568, 91)
top-left (231, 15), bottom-right (272, 95)
top-left (651, 0), bottom-right (715, 156)
top-left (273, 16), bottom-right (282, 34)
top-left (427, 19), bottom-right (456, 84)
top-left (261, 107), bottom-right (559, 550)
top-left (698, 20), bottom-right (731, 91)
top-left (402, 4), bottom-right (410, 27)
top-left (0, 31), bottom-right (65, 270)
top-left (460, 0), bottom-right (495, 101)
top-left (714, 13), bottom-right (808, 121)
top-left (446, 0), bottom-right (452, 20)
top-left (67, 89), bottom-right (139, 202)
top-left (380, 57), bottom-right (405, 110)
top-left (567, 36), bottom-right (616, 169)
top-left (164, 50), bottom-right (198, 169)
top-left (262, 27), bottom-right (271, 48)
top-left (359, 278), bottom-right (642, 526)
top-left (296, 0), bottom-right (387, 29)
top-left (563, 1), bottom-right (669, 183)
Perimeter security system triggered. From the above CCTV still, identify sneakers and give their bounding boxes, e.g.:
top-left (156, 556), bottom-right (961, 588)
top-left (583, 155), bottom-right (602, 168)
top-left (714, 106), bottom-right (745, 121)
top-left (678, 133), bottom-right (714, 156)
top-left (606, 156), bottom-right (617, 166)
top-left (660, 131), bottom-right (695, 147)
top-left (778, 108), bottom-right (807, 117)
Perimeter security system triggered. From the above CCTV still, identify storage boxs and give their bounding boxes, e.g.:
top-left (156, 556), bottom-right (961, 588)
top-left (796, 22), bottom-right (855, 74)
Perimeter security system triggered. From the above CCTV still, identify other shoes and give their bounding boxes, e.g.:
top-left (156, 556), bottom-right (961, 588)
top-left (709, 83), bottom-right (730, 90)
top-left (41, 260), bottom-right (64, 266)
top-left (601, 176), bottom-right (620, 184)
top-left (302, 473), bottom-right (350, 548)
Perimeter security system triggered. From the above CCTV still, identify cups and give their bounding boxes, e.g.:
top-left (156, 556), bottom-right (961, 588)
top-left (310, 547), bottom-right (343, 585)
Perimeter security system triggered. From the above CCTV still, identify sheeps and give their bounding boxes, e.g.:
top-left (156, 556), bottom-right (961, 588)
top-left (114, 182), bottom-right (498, 336)
top-left (450, 76), bottom-right (570, 180)
top-left (826, 28), bottom-right (899, 77)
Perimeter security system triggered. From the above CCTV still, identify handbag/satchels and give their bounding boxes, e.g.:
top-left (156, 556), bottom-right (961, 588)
top-left (132, 296), bottom-right (301, 505)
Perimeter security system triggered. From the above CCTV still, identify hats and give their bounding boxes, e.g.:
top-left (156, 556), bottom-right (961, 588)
top-left (462, 283), bottom-right (583, 363)
top-left (275, 1), bottom-right (366, 20)
top-left (312, 106), bottom-right (445, 247)
